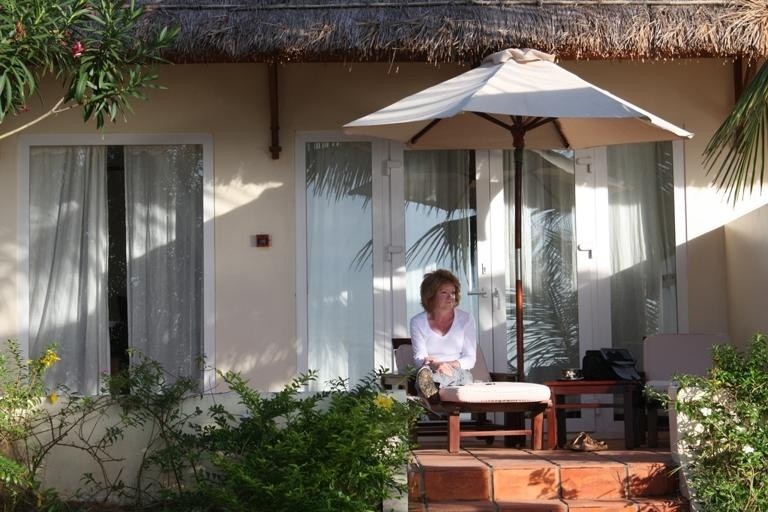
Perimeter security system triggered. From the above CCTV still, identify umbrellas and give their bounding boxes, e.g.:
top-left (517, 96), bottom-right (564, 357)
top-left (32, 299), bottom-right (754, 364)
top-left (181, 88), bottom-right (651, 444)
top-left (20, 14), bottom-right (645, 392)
top-left (340, 47), bottom-right (694, 381)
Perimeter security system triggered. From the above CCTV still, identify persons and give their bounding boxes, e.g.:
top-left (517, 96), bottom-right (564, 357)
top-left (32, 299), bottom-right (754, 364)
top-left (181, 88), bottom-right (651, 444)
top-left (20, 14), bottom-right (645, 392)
top-left (409, 268), bottom-right (478, 403)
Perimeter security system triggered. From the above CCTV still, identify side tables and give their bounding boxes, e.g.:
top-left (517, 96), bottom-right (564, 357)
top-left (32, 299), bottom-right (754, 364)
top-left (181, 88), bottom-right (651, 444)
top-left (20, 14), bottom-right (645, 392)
top-left (545, 375), bottom-right (644, 452)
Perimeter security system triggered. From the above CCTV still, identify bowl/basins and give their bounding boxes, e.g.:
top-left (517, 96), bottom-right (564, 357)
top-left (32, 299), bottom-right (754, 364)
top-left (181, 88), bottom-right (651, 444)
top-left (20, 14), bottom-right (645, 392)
top-left (563, 369), bottom-right (581, 378)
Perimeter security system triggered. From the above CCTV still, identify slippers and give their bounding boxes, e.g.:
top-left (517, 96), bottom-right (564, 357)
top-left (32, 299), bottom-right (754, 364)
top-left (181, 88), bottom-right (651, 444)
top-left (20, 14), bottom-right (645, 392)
top-left (563, 432), bottom-right (609, 452)
top-left (415, 367), bottom-right (442, 405)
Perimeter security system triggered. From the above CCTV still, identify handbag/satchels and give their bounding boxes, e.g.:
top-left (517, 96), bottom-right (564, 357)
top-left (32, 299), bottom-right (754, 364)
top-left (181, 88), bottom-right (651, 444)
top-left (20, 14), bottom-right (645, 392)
top-left (582, 349), bottom-right (642, 381)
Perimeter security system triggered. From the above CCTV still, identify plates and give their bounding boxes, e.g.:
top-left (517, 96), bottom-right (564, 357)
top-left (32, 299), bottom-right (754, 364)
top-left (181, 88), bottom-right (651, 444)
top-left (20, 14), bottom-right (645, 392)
top-left (561, 377), bottom-right (584, 381)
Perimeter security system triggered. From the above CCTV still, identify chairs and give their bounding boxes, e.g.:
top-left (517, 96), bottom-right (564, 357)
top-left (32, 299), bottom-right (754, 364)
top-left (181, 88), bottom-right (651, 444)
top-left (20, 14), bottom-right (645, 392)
top-left (642, 331), bottom-right (731, 448)
top-left (385, 334), bottom-right (552, 452)
